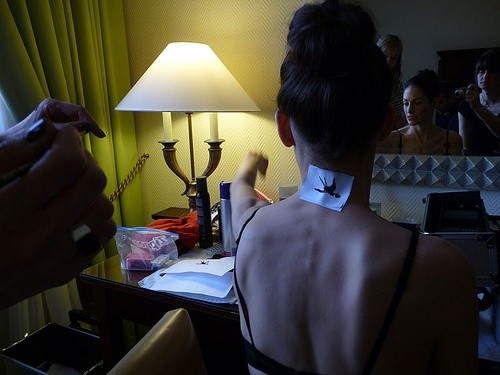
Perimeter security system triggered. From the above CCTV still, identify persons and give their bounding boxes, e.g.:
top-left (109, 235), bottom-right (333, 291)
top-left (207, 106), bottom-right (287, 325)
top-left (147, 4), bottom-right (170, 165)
top-left (0, 99), bottom-right (117, 310)
top-left (375, 68), bottom-right (463, 155)
top-left (376, 34), bottom-right (407, 129)
top-left (229, 0), bottom-right (477, 375)
top-left (457, 50), bottom-right (500, 156)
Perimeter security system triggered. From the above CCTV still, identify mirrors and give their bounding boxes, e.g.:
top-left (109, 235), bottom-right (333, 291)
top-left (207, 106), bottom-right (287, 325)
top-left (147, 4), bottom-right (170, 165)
top-left (344, 0), bottom-right (500, 192)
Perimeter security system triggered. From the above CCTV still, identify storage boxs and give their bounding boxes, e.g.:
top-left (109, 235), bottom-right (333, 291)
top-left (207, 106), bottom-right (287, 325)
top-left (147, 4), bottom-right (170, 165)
top-left (0, 322), bottom-right (105, 375)
top-left (112, 226), bottom-right (179, 271)
top-left (423, 193), bottom-right (497, 286)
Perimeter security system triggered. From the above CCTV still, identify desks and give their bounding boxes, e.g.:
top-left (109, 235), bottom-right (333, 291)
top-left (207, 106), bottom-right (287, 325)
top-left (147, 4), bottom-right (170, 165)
top-left (75, 238), bottom-right (500, 375)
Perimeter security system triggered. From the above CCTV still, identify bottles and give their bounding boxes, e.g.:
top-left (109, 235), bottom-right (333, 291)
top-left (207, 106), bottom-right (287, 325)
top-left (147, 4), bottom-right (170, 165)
top-left (278, 184), bottom-right (298, 201)
top-left (196, 175), bottom-right (213, 249)
top-left (220, 182), bottom-right (236, 257)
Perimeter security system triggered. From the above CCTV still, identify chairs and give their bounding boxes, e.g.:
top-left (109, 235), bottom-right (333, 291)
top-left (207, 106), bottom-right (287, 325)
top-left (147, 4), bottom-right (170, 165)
top-left (108, 308), bottom-right (209, 375)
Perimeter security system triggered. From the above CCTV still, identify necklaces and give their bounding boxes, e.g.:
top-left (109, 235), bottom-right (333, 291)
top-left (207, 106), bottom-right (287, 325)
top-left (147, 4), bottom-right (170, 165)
top-left (482, 95), bottom-right (494, 107)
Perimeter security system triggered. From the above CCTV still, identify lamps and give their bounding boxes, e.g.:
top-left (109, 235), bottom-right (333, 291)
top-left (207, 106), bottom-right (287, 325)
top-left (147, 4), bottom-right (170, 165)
top-left (114, 42), bottom-right (261, 212)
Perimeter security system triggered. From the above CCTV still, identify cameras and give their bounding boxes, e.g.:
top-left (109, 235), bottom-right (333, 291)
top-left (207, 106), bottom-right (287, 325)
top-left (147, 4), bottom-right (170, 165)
top-left (454, 87), bottom-right (469, 99)
